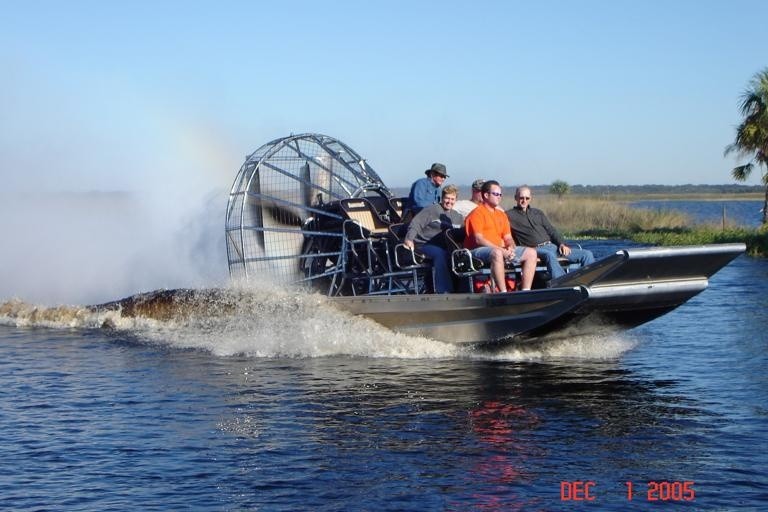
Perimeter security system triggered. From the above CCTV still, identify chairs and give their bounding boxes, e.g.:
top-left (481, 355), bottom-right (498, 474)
top-left (340, 196), bottom-right (583, 295)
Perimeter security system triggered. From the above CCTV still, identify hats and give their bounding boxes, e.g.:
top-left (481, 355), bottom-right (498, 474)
top-left (425, 162), bottom-right (450, 178)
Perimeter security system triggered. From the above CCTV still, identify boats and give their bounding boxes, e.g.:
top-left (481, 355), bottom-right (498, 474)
top-left (225, 133), bottom-right (746, 345)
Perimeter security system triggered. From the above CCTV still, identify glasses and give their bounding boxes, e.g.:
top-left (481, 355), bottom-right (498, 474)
top-left (520, 196), bottom-right (530, 200)
top-left (492, 192), bottom-right (502, 197)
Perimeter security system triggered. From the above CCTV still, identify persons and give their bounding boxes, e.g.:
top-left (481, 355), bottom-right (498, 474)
top-left (404, 163), bottom-right (595, 292)
